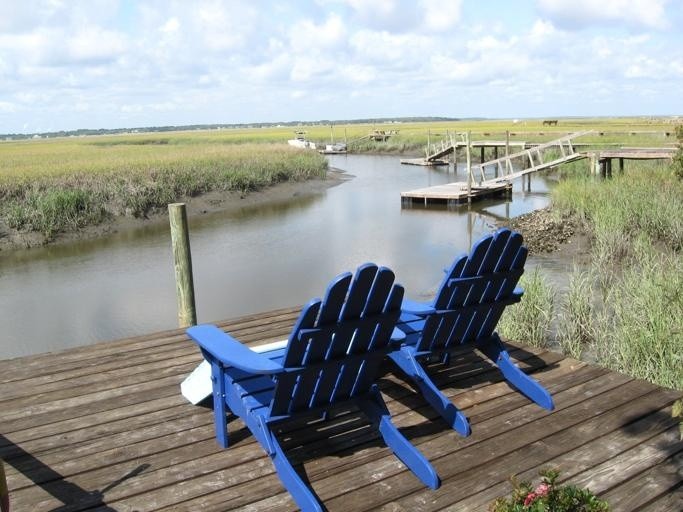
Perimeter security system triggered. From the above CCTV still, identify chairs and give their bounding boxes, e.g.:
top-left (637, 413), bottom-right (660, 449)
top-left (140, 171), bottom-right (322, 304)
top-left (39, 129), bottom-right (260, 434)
top-left (185, 263), bottom-right (440, 512)
top-left (319, 229), bottom-right (554, 437)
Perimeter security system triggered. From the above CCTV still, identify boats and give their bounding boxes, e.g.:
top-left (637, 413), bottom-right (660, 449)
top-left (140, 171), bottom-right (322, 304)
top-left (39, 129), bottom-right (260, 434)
top-left (318, 142), bottom-right (349, 156)
top-left (285, 135), bottom-right (316, 151)
top-left (295, 128), bottom-right (307, 135)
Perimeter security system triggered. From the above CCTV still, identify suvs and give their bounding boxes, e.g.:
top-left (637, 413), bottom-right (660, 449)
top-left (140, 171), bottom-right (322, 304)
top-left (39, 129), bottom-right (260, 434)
top-left (369, 130), bottom-right (391, 141)
